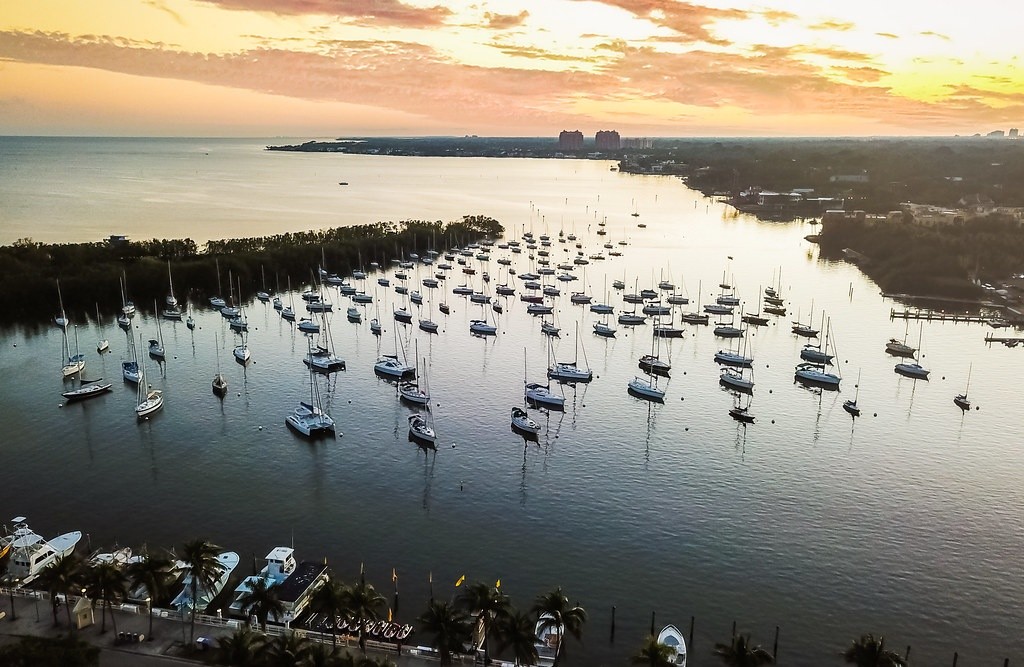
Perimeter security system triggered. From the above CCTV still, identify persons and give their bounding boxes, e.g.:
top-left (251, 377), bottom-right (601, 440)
top-left (396, 640), bottom-right (402, 655)
top-left (55, 596), bottom-right (60, 607)
top-left (344, 631), bottom-right (367, 655)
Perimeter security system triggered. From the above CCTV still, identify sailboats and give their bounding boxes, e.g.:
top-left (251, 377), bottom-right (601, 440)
top-left (843, 367), bottom-right (861, 414)
top-left (407, 357), bottom-right (439, 443)
top-left (524, 328), bottom-right (567, 409)
top-left (212, 331), bottom-right (228, 395)
top-left (510, 347), bottom-right (542, 435)
top-left (894, 320), bottom-right (930, 378)
top-left (728, 373), bottom-right (756, 422)
top-left (61, 332), bottom-right (86, 378)
top-left (114, 193), bottom-right (918, 387)
top-left (284, 334), bottom-right (337, 439)
top-left (61, 324), bottom-right (113, 403)
top-left (955, 361), bottom-right (972, 406)
top-left (627, 316), bottom-right (665, 400)
top-left (96, 302), bottom-right (110, 352)
top-left (61, 309), bottom-right (87, 362)
top-left (52, 277), bottom-right (69, 327)
top-left (133, 332), bottom-right (165, 418)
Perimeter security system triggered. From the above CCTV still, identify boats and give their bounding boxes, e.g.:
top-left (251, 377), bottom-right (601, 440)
top-left (461, 585), bottom-right (502, 649)
top-left (656, 623), bottom-right (686, 667)
top-left (0, 516), bottom-right (240, 616)
top-left (529, 608), bottom-right (565, 667)
top-left (228, 546), bottom-right (297, 622)
top-left (266, 560), bottom-right (330, 626)
